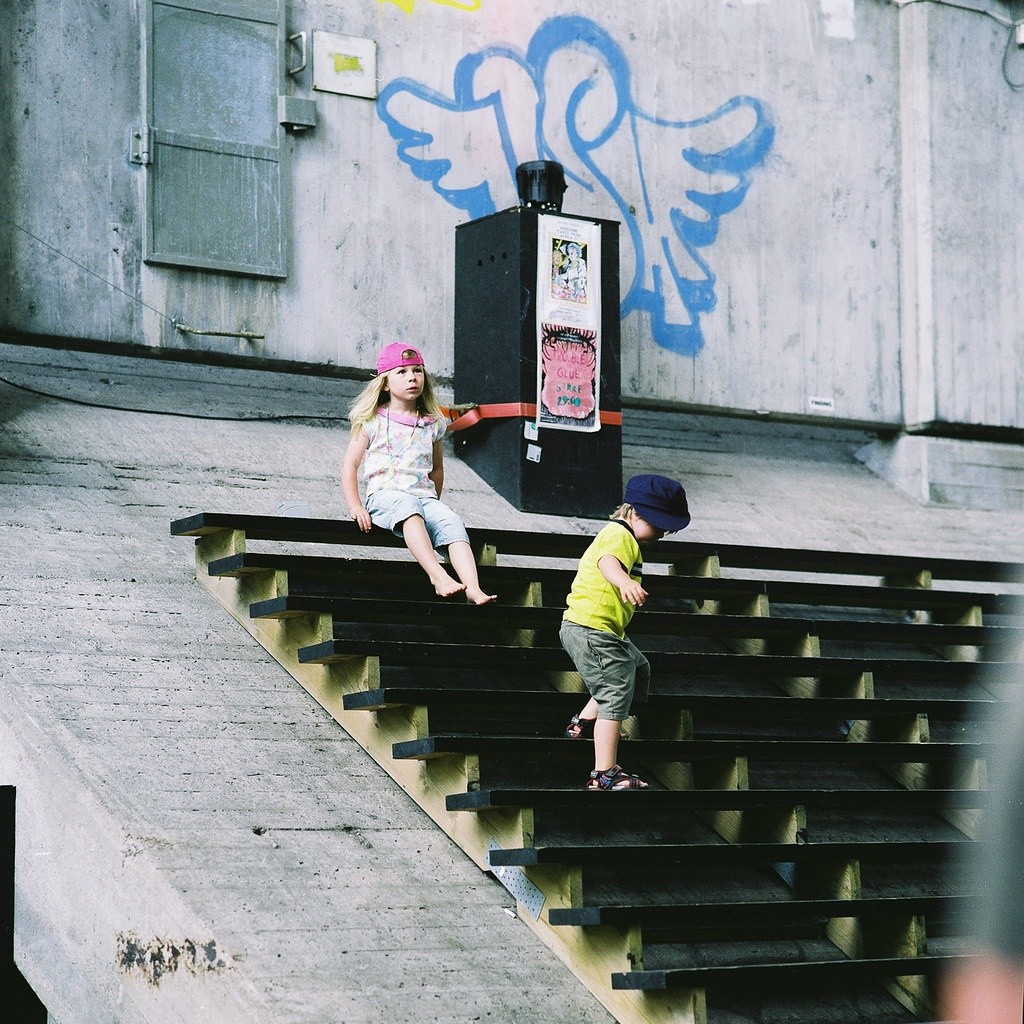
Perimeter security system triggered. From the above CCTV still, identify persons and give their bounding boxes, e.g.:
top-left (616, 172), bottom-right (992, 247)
top-left (340, 341), bottom-right (499, 606)
top-left (560, 474), bottom-right (693, 793)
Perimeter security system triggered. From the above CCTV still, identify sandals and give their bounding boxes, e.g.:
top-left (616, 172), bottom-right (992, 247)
top-left (565, 714), bottom-right (629, 741)
top-left (585, 764), bottom-right (650, 791)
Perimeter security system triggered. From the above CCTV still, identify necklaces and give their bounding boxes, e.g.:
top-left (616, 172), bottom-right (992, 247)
top-left (385, 404), bottom-right (419, 459)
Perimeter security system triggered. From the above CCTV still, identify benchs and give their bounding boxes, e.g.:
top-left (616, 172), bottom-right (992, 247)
top-left (169, 511), bottom-right (1024, 1024)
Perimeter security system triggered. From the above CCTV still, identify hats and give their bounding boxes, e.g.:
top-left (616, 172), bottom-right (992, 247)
top-left (623, 474), bottom-right (691, 531)
top-left (376, 341), bottom-right (424, 375)
